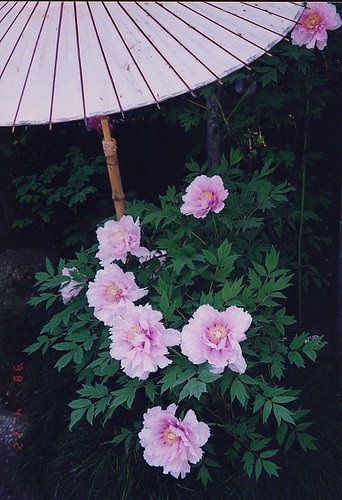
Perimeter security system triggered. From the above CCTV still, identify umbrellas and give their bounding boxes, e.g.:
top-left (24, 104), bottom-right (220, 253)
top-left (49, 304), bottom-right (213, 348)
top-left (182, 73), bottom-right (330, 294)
top-left (0, 1), bottom-right (308, 223)
